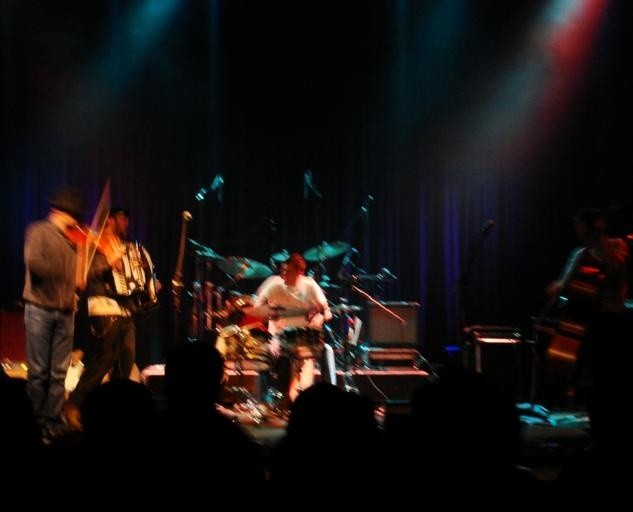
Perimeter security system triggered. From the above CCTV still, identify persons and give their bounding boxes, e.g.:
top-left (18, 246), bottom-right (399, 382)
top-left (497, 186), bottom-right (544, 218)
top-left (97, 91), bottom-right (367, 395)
top-left (23, 195), bottom-right (86, 443)
top-left (546, 210), bottom-right (632, 321)
top-left (253, 253), bottom-right (333, 405)
top-left (1, 344), bottom-right (633, 511)
top-left (60, 205), bottom-right (162, 433)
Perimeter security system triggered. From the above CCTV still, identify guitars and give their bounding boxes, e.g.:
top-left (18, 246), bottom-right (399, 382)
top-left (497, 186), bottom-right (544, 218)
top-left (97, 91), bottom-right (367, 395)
top-left (535, 264), bottom-right (633, 385)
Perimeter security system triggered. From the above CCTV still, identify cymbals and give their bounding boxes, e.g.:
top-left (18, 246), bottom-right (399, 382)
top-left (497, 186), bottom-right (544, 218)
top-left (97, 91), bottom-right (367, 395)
top-left (304, 241), bottom-right (347, 263)
top-left (217, 257), bottom-right (272, 279)
top-left (187, 238), bottom-right (225, 262)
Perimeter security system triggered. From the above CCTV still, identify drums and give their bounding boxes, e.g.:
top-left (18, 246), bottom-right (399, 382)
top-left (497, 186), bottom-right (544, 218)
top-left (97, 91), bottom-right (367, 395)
top-left (203, 287), bottom-right (243, 333)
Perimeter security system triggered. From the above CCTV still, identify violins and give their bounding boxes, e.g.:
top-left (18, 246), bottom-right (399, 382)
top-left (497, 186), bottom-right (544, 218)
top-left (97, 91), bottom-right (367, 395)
top-left (66, 226), bottom-right (124, 271)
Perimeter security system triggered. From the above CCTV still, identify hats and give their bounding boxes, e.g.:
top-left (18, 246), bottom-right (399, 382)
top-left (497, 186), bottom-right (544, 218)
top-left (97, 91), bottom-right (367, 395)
top-left (45, 186), bottom-right (88, 218)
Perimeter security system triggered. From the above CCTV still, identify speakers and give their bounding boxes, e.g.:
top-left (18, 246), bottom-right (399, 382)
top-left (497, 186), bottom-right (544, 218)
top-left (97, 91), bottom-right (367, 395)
top-left (462, 324), bottom-right (537, 403)
top-left (366, 301), bottom-right (421, 347)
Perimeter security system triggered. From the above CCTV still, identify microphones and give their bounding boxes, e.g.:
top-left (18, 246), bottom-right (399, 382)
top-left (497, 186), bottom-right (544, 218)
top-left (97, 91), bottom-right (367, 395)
top-left (336, 248), bottom-right (356, 276)
top-left (303, 173), bottom-right (312, 198)
